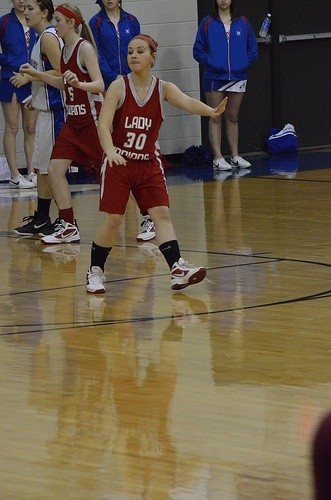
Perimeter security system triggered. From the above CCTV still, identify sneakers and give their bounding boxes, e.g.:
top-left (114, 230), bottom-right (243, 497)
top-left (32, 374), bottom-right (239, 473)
top-left (229, 155), bottom-right (252, 168)
top-left (83, 264), bottom-right (109, 296)
top-left (12, 209), bottom-right (53, 237)
top-left (135, 214), bottom-right (157, 242)
top-left (168, 256), bottom-right (208, 292)
top-left (40, 217), bottom-right (82, 246)
top-left (38, 212), bottom-right (60, 237)
top-left (24, 172), bottom-right (38, 187)
top-left (7, 173), bottom-right (36, 189)
top-left (211, 157), bottom-right (232, 172)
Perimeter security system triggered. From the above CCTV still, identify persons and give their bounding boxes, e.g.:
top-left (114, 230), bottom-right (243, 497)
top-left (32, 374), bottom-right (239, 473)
top-left (193, 0), bottom-right (258, 170)
top-left (9, 0), bottom-right (81, 237)
top-left (88, 0), bottom-right (141, 92)
top-left (85, 34), bottom-right (229, 294)
top-left (0, 0), bottom-right (39, 189)
top-left (19, 2), bottom-right (155, 245)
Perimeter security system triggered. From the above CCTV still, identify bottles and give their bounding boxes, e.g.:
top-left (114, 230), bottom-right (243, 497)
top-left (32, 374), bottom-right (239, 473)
top-left (258, 13), bottom-right (271, 37)
